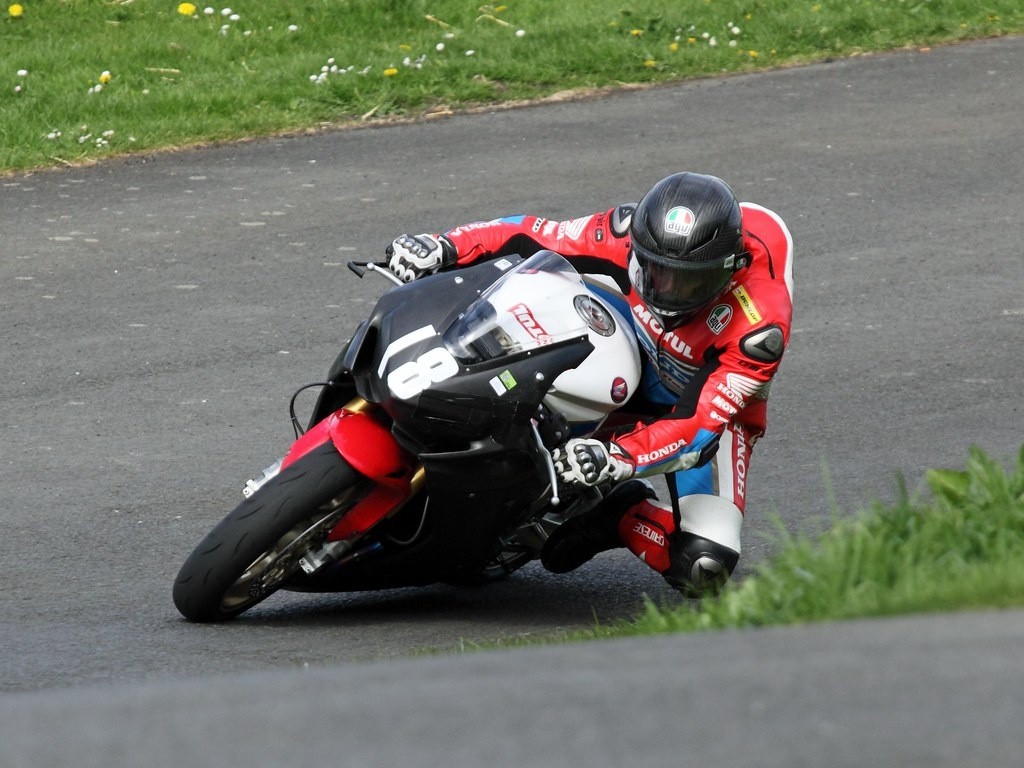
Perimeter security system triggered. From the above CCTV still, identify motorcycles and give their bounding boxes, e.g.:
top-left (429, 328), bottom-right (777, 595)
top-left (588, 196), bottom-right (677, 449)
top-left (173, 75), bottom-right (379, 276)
top-left (173, 244), bottom-right (645, 625)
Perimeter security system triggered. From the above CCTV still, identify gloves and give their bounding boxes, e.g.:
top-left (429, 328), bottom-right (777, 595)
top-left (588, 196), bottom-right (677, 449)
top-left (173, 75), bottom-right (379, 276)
top-left (384, 232), bottom-right (460, 283)
top-left (551, 438), bottom-right (635, 490)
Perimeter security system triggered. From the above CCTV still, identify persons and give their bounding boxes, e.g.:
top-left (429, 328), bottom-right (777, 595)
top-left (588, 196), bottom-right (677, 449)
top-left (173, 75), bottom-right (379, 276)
top-left (386, 172), bottom-right (793, 601)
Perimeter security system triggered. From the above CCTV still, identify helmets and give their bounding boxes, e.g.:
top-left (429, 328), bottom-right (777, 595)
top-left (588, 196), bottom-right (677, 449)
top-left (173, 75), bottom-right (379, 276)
top-left (628, 172), bottom-right (752, 333)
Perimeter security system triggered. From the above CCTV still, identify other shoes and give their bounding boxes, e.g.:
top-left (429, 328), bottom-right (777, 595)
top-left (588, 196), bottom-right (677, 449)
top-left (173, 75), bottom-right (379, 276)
top-left (541, 478), bottom-right (659, 574)
